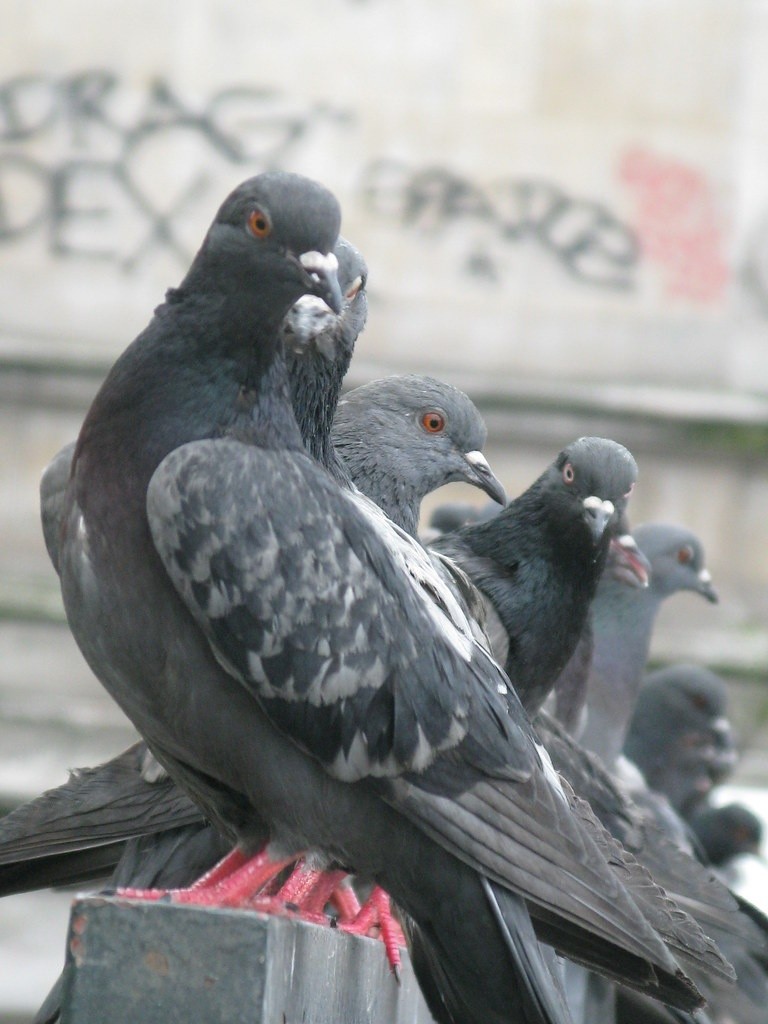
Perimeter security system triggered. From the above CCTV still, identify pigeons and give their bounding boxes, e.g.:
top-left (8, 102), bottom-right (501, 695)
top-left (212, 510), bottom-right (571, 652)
top-left (1, 165), bottom-right (768, 1024)
